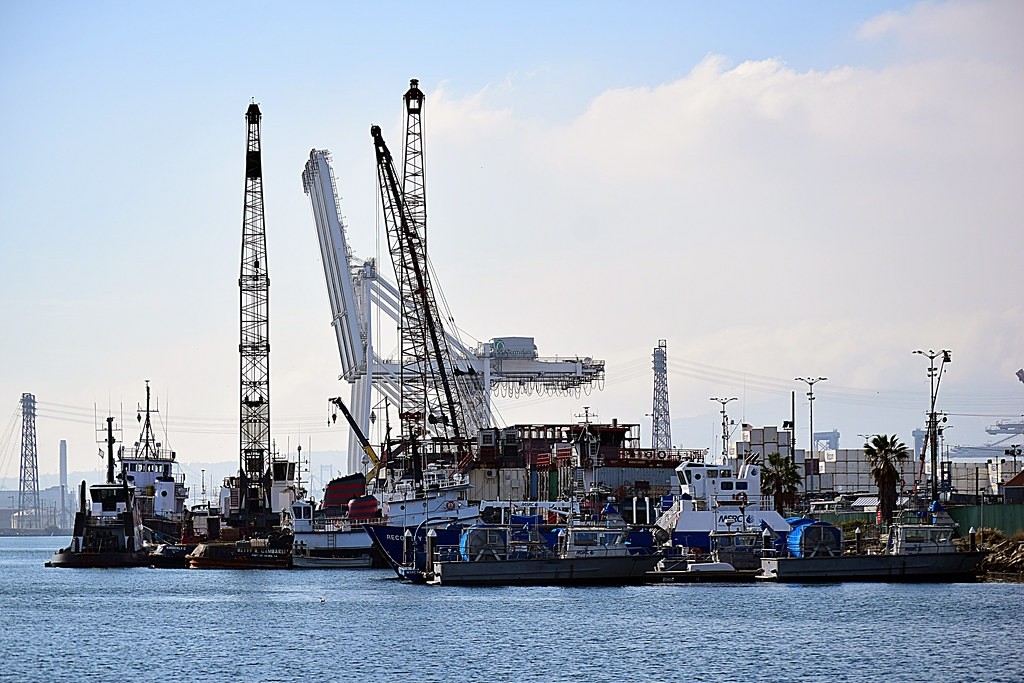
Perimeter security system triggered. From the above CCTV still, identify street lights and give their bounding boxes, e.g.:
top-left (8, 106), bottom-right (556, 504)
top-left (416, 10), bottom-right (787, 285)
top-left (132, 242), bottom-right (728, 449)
top-left (793, 376), bottom-right (829, 495)
top-left (912, 349), bottom-right (953, 501)
top-left (709, 396), bottom-right (740, 455)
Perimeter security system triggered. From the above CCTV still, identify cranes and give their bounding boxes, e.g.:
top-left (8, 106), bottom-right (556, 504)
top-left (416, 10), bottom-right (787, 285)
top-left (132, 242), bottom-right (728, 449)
top-left (229, 105), bottom-right (280, 537)
top-left (370, 78), bottom-right (508, 488)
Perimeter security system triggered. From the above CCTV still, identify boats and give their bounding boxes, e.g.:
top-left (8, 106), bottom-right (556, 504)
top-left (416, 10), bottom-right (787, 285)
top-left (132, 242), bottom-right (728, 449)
top-left (45, 375), bottom-right (989, 587)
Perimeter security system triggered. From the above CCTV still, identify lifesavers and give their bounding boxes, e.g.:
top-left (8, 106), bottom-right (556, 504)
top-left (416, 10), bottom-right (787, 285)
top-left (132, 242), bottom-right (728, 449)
top-left (586, 458), bottom-right (594, 466)
top-left (645, 452), bottom-right (652, 458)
top-left (657, 450), bottom-right (667, 459)
top-left (446, 501), bottom-right (455, 510)
